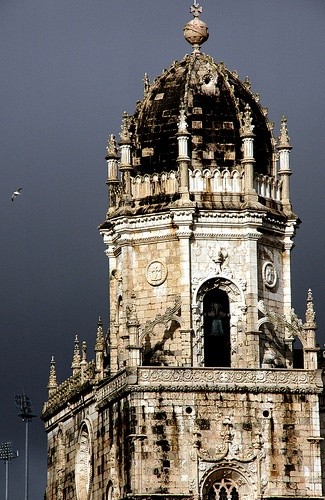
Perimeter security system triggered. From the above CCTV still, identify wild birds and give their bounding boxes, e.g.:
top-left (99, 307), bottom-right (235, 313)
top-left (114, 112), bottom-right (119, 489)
top-left (11, 187), bottom-right (23, 202)
